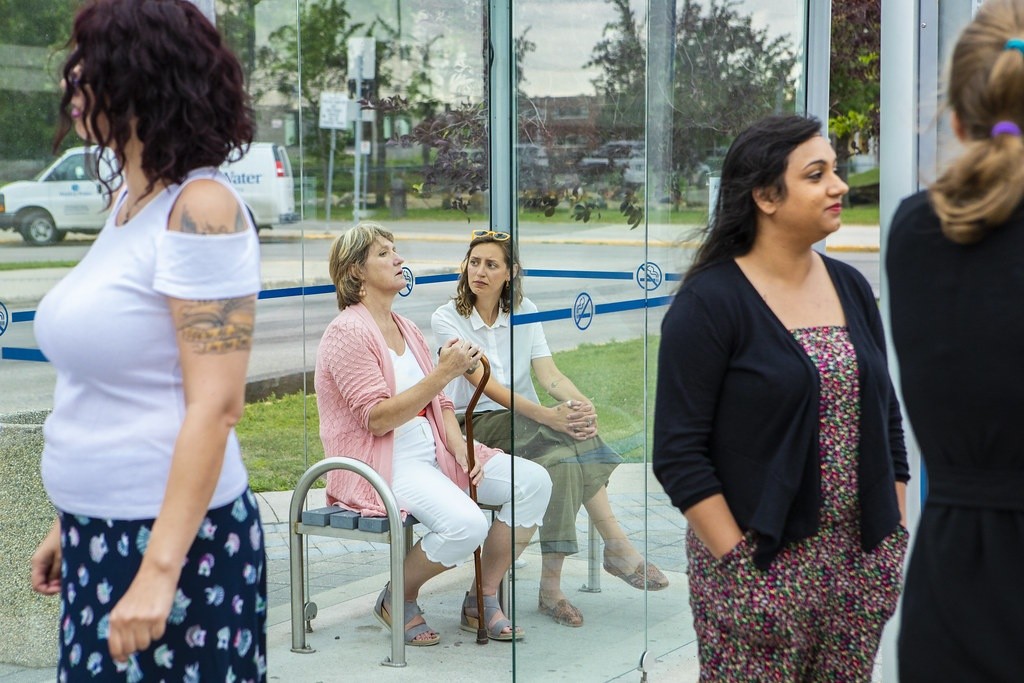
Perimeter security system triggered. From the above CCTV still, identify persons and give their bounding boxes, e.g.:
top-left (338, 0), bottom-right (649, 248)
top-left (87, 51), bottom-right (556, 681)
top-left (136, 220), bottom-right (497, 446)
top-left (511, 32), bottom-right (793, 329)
top-left (315, 220), bottom-right (554, 648)
top-left (28, 0), bottom-right (267, 683)
top-left (429, 228), bottom-right (671, 628)
top-left (651, 114), bottom-right (912, 683)
top-left (881, 0), bottom-right (1024, 683)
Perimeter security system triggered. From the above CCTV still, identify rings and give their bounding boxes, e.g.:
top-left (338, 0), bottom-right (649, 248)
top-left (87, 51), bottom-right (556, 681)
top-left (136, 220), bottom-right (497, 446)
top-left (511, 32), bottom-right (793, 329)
top-left (587, 421), bottom-right (592, 426)
top-left (566, 400), bottom-right (572, 408)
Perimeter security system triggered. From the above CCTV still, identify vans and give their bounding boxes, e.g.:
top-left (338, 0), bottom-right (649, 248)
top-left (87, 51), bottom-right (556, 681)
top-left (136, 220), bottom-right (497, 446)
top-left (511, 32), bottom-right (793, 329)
top-left (0, 136), bottom-right (299, 245)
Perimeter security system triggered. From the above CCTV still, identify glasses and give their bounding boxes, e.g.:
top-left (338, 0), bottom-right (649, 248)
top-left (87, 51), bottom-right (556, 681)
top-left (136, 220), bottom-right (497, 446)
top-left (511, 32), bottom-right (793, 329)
top-left (472, 229), bottom-right (510, 241)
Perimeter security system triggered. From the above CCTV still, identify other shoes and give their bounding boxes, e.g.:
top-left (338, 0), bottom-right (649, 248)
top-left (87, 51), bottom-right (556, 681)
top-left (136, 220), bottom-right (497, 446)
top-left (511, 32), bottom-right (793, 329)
top-left (603, 547), bottom-right (669, 591)
top-left (537, 589), bottom-right (584, 627)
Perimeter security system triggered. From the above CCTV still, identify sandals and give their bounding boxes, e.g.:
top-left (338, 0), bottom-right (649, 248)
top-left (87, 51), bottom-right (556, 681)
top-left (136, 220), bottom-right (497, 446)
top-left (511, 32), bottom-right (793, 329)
top-left (460, 592), bottom-right (524, 640)
top-left (372, 580), bottom-right (440, 646)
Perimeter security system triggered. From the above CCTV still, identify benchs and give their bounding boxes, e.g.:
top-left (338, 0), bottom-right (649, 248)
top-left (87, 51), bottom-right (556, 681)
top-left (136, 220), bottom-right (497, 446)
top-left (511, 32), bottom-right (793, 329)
top-left (284, 457), bottom-right (604, 668)
